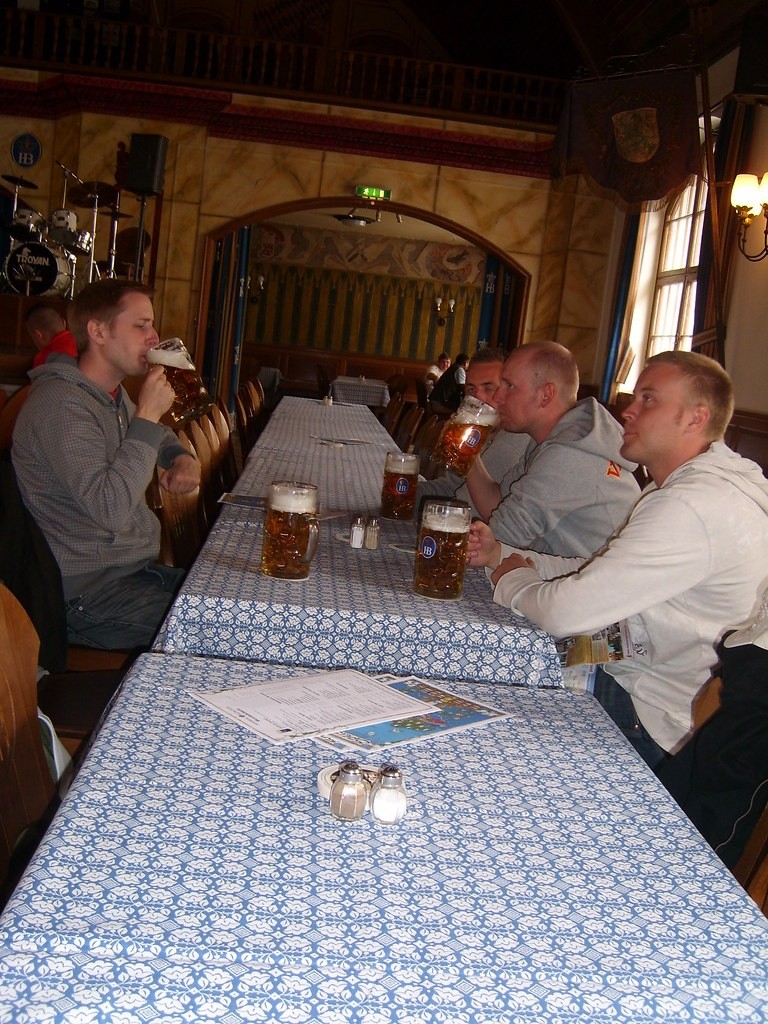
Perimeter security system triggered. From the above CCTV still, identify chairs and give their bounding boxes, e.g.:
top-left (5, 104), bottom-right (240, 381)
top-left (0, 373), bottom-right (268, 913)
top-left (314, 362), bottom-right (331, 399)
top-left (381, 374), bottom-right (448, 481)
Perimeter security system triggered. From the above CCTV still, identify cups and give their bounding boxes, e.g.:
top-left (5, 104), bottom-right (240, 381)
top-left (414, 499), bottom-right (479, 599)
top-left (430, 393), bottom-right (502, 478)
top-left (380, 452), bottom-right (420, 521)
top-left (146, 338), bottom-right (213, 424)
top-left (260, 480), bottom-right (318, 579)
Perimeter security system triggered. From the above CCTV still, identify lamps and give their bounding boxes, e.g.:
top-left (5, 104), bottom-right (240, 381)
top-left (247, 273), bottom-right (265, 304)
top-left (730, 171), bottom-right (768, 262)
top-left (435, 297), bottom-right (456, 326)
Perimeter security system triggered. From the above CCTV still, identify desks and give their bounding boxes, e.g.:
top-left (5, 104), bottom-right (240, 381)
top-left (329, 374), bottom-right (391, 407)
top-left (143, 394), bottom-right (560, 688)
top-left (0, 651), bottom-right (768, 1024)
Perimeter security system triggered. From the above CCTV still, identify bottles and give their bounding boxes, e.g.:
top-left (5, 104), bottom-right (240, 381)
top-left (330, 762), bottom-right (367, 822)
top-left (369, 766), bottom-right (407, 825)
top-left (350, 514), bottom-right (380, 549)
top-left (324, 395), bottom-right (333, 406)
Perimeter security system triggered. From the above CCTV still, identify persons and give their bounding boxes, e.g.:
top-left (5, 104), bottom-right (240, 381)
top-left (11, 280), bottom-right (200, 650)
top-left (424, 354), bottom-right (469, 415)
top-left (466, 350), bottom-right (768, 768)
top-left (26, 305), bottom-right (78, 370)
top-left (441, 340), bottom-right (641, 558)
top-left (412, 348), bottom-right (530, 522)
